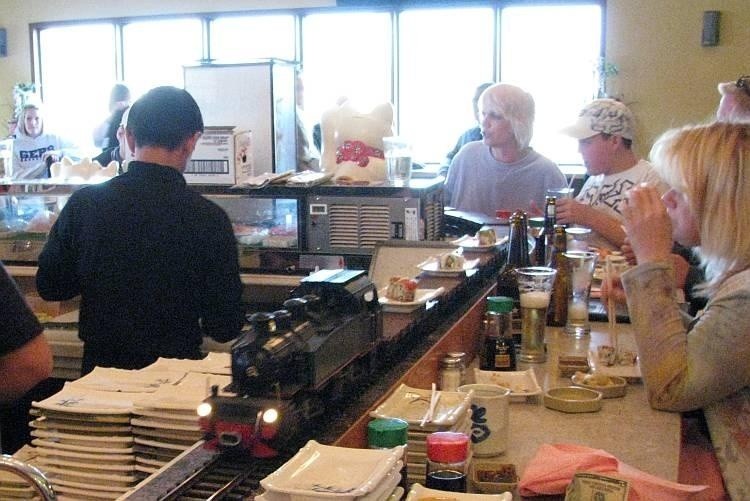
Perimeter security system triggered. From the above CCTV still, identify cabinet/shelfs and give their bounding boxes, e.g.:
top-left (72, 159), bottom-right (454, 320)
top-left (0, 179), bottom-right (303, 277)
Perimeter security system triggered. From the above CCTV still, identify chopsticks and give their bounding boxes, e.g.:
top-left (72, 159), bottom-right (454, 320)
top-left (606, 256), bottom-right (618, 353)
top-left (420, 380), bottom-right (443, 430)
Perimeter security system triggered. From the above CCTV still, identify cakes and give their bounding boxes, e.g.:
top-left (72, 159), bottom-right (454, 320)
top-left (599, 345), bottom-right (638, 367)
top-left (439, 253), bottom-right (462, 270)
top-left (480, 228), bottom-right (496, 246)
top-left (385, 276), bottom-right (417, 303)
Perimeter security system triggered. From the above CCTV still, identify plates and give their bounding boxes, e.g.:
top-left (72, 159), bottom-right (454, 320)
top-left (368, 383), bottom-right (477, 489)
top-left (61, 365), bottom-right (189, 392)
top-left (377, 283), bottom-right (446, 314)
top-left (254, 438), bottom-right (408, 500)
top-left (472, 365), bottom-right (544, 405)
top-left (134, 356), bottom-right (196, 376)
top-left (174, 375), bottom-right (232, 395)
top-left (541, 384), bottom-right (606, 415)
top-left (588, 344), bottom-right (645, 383)
top-left (190, 351), bottom-right (237, 374)
top-left (446, 232), bottom-right (513, 253)
top-left (415, 255), bottom-right (481, 278)
top-left (404, 481), bottom-right (517, 501)
top-left (26, 379), bottom-right (134, 501)
top-left (572, 376), bottom-right (627, 400)
top-left (129, 383), bottom-right (207, 483)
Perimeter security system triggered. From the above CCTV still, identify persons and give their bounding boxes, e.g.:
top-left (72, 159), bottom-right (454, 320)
top-left (37, 86), bottom-right (244, 376)
top-left (296, 68), bottom-right (320, 172)
top-left (441, 83), bottom-right (497, 175)
top-left (7, 104), bottom-right (70, 183)
top-left (93, 86), bottom-right (128, 149)
top-left (442, 85), bottom-right (570, 226)
top-left (601, 120), bottom-right (750, 500)
top-left (88, 106), bottom-right (136, 173)
top-left (527, 98), bottom-right (659, 253)
top-left (622, 80), bottom-right (749, 307)
top-left (1, 263), bottom-right (61, 455)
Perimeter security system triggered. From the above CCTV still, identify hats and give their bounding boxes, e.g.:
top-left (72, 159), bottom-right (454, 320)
top-left (559, 97), bottom-right (637, 148)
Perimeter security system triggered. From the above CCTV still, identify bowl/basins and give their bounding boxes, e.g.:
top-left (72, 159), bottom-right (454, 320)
top-left (558, 356), bottom-right (590, 379)
top-left (472, 463), bottom-right (517, 495)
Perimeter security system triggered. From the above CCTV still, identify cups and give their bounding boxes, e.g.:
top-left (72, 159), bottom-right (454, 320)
top-left (546, 186), bottom-right (574, 201)
top-left (381, 136), bottom-right (416, 183)
top-left (561, 246), bottom-right (600, 336)
top-left (514, 266), bottom-right (556, 367)
top-left (458, 382), bottom-right (513, 460)
top-left (565, 228), bottom-right (593, 251)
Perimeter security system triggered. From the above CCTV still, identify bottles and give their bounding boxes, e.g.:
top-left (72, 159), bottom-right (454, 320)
top-left (439, 358), bottom-right (461, 393)
top-left (367, 419), bottom-right (408, 493)
top-left (517, 212), bottom-right (529, 223)
top-left (480, 297), bottom-right (517, 371)
top-left (498, 209), bottom-right (512, 221)
top-left (427, 432), bottom-right (468, 491)
top-left (536, 195), bottom-right (555, 267)
top-left (449, 352), bottom-right (466, 388)
top-left (530, 217), bottom-right (546, 241)
top-left (497, 219), bottom-right (528, 351)
top-left (546, 224), bottom-right (572, 327)
